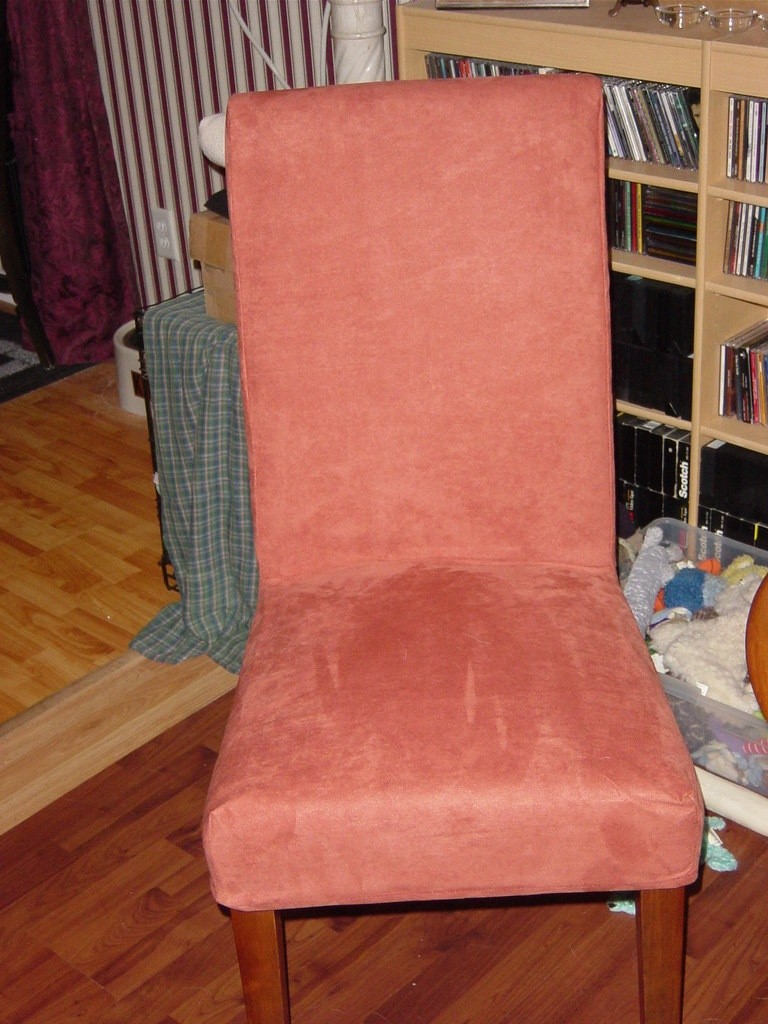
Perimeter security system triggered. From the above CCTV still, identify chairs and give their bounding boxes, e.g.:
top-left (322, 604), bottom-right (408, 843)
top-left (200, 71), bottom-right (707, 1024)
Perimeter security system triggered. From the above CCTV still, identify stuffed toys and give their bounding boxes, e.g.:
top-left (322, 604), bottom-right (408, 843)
top-left (624, 527), bottom-right (768, 792)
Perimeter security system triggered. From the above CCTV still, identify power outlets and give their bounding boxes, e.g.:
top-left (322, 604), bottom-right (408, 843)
top-left (149, 208), bottom-right (180, 261)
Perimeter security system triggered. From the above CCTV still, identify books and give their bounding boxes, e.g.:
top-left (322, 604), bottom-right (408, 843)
top-left (717, 319), bottom-right (768, 428)
top-left (698, 439), bottom-right (768, 569)
top-left (722, 200), bottom-right (768, 282)
top-left (726, 95), bottom-right (768, 184)
top-left (613, 412), bottom-right (692, 555)
top-left (423, 54), bottom-right (702, 172)
top-left (608, 272), bottom-right (694, 422)
top-left (607, 179), bottom-right (696, 267)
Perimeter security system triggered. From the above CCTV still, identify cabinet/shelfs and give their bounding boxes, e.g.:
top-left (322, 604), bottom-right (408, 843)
top-left (394, 0), bottom-right (768, 549)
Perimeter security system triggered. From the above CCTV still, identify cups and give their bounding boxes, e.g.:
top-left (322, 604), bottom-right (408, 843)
top-left (656, 4), bottom-right (706, 28)
top-left (758, 12), bottom-right (768, 32)
top-left (705, 7), bottom-right (758, 31)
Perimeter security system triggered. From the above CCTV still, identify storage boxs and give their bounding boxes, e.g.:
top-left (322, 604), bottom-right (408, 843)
top-left (186, 209), bottom-right (235, 326)
top-left (613, 515), bottom-right (768, 837)
